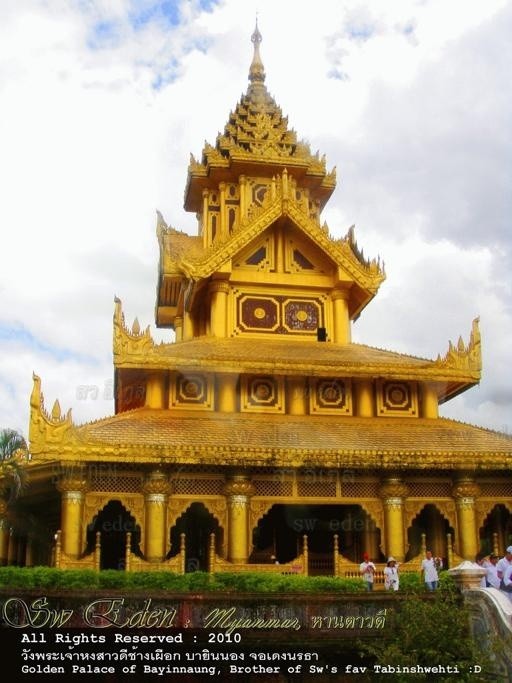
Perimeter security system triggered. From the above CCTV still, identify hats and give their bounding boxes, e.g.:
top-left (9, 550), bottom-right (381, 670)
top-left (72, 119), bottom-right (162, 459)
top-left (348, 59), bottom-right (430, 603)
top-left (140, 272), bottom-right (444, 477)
top-left (506, 546), bottom-right (512, 552)
top-left (387, 556), bottom-right (397, 563)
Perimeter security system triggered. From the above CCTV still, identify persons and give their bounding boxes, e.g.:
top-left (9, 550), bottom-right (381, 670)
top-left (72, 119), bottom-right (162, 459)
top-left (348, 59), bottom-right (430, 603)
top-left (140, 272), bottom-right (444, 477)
top-left (476, 546), bottom-right (512, 604)
top-left (420, 550), bottom-right (442, 592)
top-left (270, 554), bottom-right (280, 564)
top-left (383, 556), bottom-right (400, 592)
top-left (359, 551), bottom-right (377, 591)
